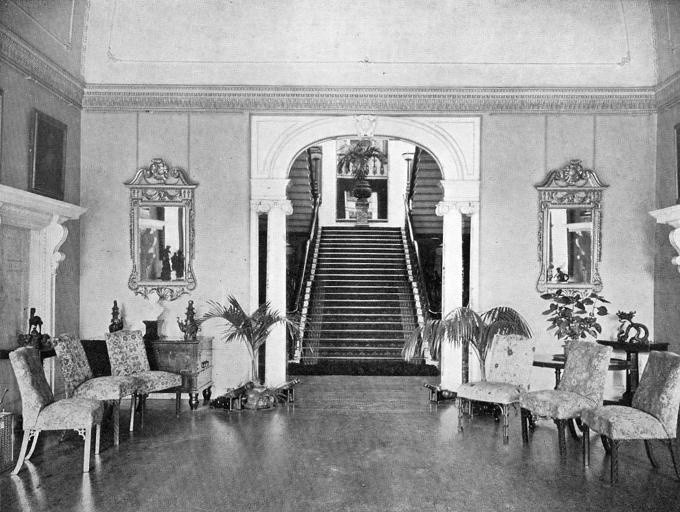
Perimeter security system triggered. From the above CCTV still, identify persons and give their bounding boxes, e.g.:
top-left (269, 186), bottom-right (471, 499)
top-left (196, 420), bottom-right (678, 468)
top-left (160, 245), bottom-right (172, 272)
top-left (176, 249), bottom-right (185, 274)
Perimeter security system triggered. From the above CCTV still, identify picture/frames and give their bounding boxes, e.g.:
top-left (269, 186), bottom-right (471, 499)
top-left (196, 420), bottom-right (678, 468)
top-left (25, 109), bottom-right (72, 202)
top-left (673, 121), bottom-right (680, 204)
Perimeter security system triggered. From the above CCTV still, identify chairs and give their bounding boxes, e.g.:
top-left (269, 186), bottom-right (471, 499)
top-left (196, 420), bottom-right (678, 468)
top-left (49, 330), bottom-right (139, 448)
top-left (518, 332), bottom-right (615, 466)
top-left (579, 346), bottom-right (679, 485)
top-left (104, 327), bottom-right (185, 430)
top-left (4, 345), bottom-right (113, 481)
top-left (456, 329), bottom-right (539, 445)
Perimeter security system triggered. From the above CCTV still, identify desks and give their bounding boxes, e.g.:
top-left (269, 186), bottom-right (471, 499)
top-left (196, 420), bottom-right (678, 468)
top-left (4, 340), bottom-right (80, 365)
top-left (595, 336), bottom-right (671, 402)
top-left (527, 353), bottom-right (632, 400)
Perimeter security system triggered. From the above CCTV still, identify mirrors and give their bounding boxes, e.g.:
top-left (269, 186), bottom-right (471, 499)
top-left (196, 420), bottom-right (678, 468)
top-left (530, 154), bottom-right (610, 303)
top-left (122, 157), bottom-right (199, 304)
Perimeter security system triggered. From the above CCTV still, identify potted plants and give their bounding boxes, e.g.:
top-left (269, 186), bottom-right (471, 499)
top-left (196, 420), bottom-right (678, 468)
top-left (538, 288), bottom-right (612, 361)
top-left (335, 140), bottom-right (389, 200)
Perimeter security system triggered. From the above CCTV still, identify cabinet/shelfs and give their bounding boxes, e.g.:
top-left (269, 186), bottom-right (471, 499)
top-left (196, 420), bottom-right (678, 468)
top-left (78, 333), bottom-right (218, 410)
